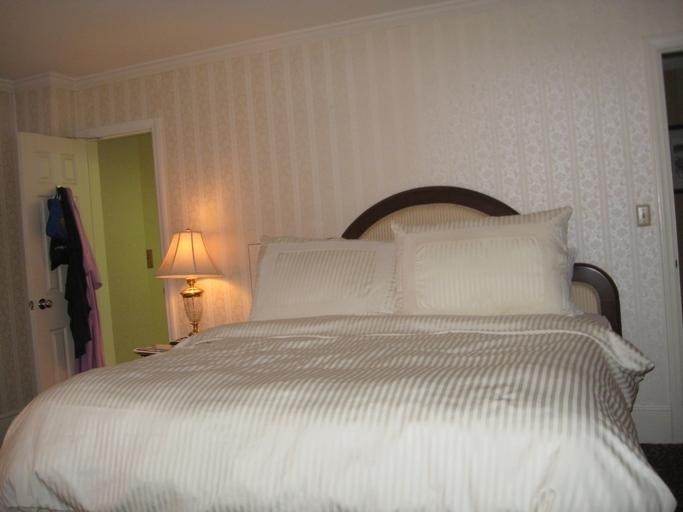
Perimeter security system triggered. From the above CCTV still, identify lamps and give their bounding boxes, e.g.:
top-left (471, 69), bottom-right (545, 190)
top-left (153, 230), bottom-right (225, 344)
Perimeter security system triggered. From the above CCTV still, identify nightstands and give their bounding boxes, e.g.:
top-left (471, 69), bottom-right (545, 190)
top-left (133, 344), bottom-right (172, 358)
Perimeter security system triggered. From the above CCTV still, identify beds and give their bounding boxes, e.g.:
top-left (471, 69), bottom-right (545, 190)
top-left (0, 184), bottom-right (677, 512)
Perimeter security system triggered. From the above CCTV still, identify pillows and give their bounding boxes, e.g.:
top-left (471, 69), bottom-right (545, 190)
top-left (391, 205), bottom-right (577, 316)
top-left (250, 238), bottom-right (394, 316)
